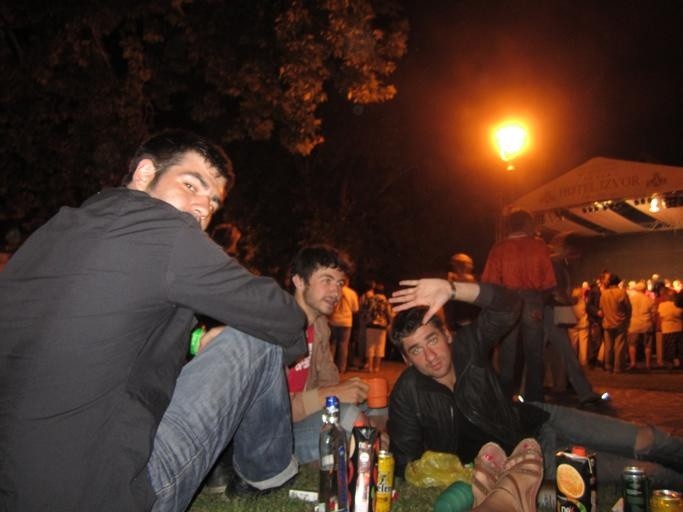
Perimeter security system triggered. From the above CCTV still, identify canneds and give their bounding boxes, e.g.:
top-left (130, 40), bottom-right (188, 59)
top-left (650, 489), bottom-right (682, 512)
top-left (620, 464), bottom-right (648, 511)
top-left (374, 450), bottom-right (395, 512)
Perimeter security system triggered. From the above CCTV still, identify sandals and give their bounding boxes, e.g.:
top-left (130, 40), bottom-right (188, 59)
top-left (472, 437), bottom-right (543, 512)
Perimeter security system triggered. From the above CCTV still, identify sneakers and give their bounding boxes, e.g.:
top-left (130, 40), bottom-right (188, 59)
top-left (205, 463), bottom-right (295, 501)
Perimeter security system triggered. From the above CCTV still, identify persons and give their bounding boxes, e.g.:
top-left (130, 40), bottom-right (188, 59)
top-left (285, 212), bottom-right (683, 511)
top-left (0, 127), bottom-right (310, 512)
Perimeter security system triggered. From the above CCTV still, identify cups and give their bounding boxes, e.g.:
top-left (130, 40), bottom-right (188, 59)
top-left (434, 481), bottom-right (474, 512)
top-left (368, 378), bottom-right (389, 409)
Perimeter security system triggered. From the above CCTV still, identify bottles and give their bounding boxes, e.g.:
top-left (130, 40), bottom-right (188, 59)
top-left (320, 396), bottom-right (347, 511)
